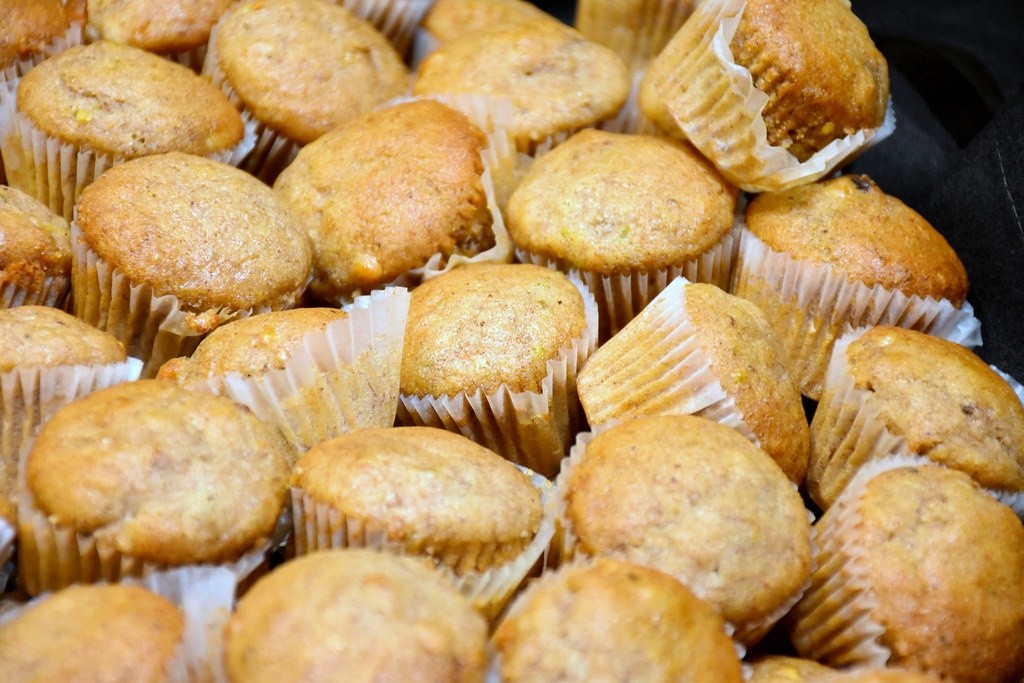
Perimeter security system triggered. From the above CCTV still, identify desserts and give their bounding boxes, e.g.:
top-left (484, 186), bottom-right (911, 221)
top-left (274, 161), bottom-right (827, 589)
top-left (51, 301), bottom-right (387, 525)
top-left (0, 0), bottom-right (1024, 679)
